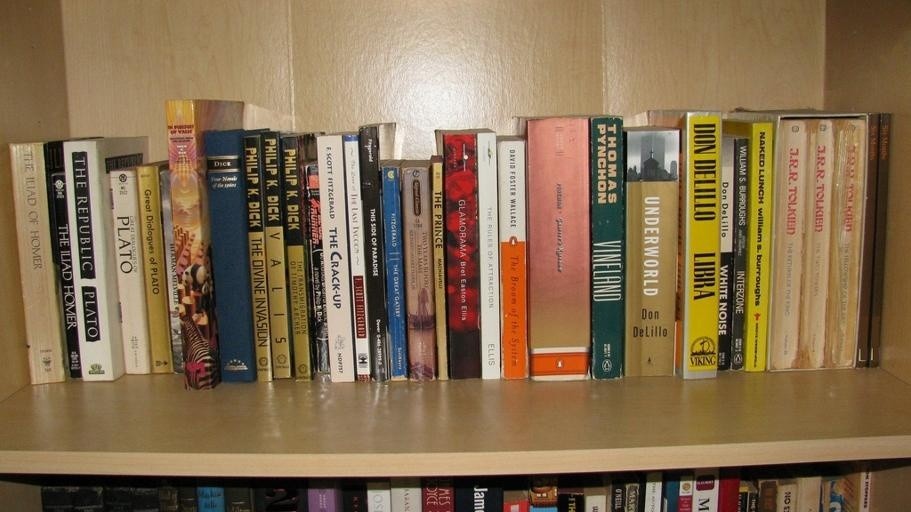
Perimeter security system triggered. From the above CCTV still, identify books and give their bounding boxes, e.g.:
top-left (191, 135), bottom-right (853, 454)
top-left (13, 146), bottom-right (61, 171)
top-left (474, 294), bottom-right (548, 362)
top-left (41, 461), bottom-right (879, 512)
top-left (7, 100), bottom-right (894, 393)
